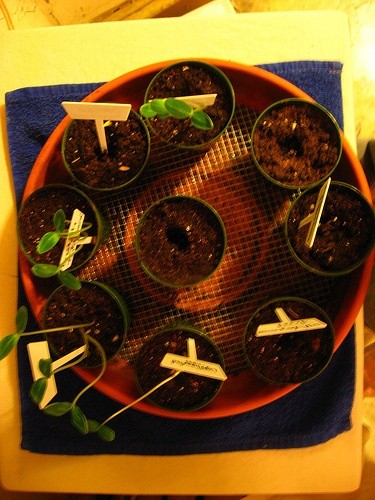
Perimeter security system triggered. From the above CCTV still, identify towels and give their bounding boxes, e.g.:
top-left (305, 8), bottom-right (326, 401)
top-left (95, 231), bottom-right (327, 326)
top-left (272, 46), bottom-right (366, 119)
top-left (4, 60), bottom-right (358, 458)
